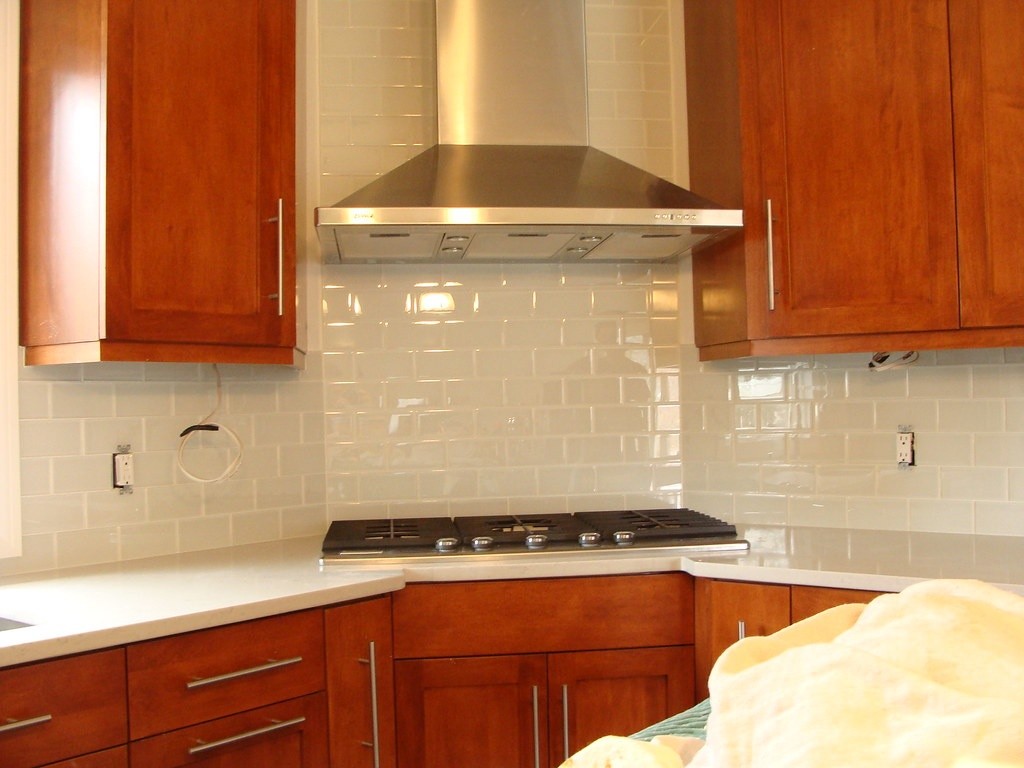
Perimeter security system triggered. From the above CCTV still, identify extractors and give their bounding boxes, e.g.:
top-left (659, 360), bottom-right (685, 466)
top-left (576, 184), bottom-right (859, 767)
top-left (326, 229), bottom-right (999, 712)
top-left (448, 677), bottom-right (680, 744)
top-left (314, 0), bottom-right (743, 263)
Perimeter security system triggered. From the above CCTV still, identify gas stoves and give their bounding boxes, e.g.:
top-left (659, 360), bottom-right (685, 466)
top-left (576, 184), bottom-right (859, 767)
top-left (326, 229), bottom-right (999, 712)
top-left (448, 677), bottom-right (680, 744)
top-left (318, 508), bottom-right (748, 567)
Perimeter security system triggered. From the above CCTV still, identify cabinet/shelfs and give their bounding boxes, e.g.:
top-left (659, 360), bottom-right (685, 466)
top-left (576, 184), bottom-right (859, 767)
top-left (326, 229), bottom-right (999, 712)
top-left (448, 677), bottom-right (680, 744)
top-left (691, 1), bottom-right (1024, 362)
top-left (16, 0), bottom-right (297, 366)
top-left (0, 588), bottom-right (397, 768)
top-left (390, 570), bottom-right (696, 768)
top-left (695, 576), bottom-right (900, 707)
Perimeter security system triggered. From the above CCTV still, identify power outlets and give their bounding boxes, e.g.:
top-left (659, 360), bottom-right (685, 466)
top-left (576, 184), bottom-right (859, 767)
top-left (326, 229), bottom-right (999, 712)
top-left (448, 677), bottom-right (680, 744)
top-left (896, 432), bottom-right (915, 463)
